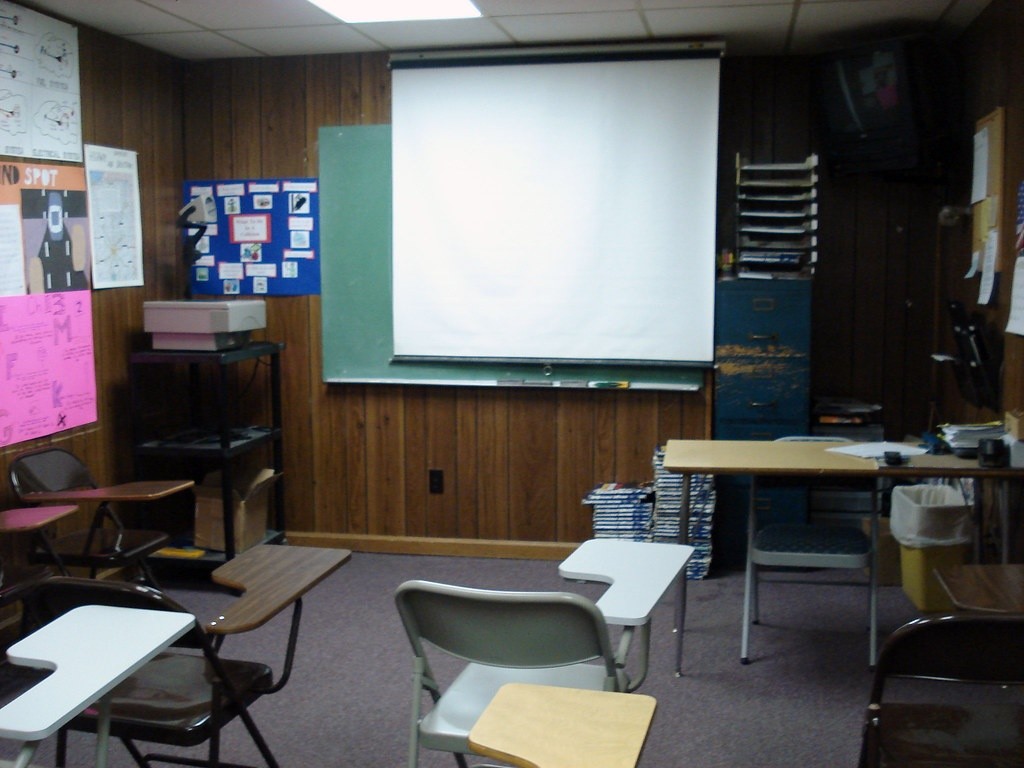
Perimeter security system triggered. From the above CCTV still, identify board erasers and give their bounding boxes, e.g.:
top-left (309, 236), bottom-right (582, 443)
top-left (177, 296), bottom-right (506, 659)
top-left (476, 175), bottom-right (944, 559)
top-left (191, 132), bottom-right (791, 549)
top-left (588, 381), bottom-right (629, 389)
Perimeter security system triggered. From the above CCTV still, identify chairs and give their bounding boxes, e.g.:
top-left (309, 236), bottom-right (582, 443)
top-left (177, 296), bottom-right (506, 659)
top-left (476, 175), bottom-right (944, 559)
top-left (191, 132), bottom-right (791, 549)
top-left (854, 562), bottom-right (1024, 768)
top-left (37, 544), bottom-right (352, 768)
top-left (0, 506), bottom-right (76, 610)
top-left (390, 537), bottom-right (695, 768)
top-left (0, 603), bottom-right (196, 768)
top-left (740, 436), bottom-right (880, 671)
top-left (8, 447), bottom-right (196, 595)
top-left (467, 683), bottom-right (656, 768)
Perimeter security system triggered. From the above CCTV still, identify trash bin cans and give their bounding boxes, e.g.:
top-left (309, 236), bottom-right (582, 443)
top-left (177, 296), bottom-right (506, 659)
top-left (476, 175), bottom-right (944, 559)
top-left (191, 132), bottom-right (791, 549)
top-left (892, 484), bottom-right (976, 613)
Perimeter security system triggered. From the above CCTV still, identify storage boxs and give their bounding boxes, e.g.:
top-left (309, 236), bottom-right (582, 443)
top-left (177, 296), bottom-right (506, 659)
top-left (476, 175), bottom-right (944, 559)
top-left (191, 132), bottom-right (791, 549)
top-left (185, 465), bottom-right (284, 554)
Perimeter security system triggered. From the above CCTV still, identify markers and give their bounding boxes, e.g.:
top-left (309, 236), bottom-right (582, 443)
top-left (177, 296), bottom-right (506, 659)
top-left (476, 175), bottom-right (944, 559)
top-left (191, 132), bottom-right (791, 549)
top-left (496, 379), bottom-right (588, 386)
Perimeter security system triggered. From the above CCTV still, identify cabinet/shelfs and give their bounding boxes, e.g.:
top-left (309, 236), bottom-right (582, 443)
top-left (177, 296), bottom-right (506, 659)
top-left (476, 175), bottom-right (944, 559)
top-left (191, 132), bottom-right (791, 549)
top-left (126, 338), bottom-right (287, 563)
top-left (708, 278), bottom-right (814, 571)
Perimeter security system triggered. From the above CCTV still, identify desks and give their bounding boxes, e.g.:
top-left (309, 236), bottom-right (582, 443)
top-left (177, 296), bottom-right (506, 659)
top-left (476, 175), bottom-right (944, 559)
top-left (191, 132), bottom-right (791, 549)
top-left (658, 436), bottom-right (1023, 678)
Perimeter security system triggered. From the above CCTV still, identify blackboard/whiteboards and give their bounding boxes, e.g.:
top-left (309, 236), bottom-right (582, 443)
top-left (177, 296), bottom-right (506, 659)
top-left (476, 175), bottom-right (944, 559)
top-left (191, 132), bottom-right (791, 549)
top-left (316, 123), bottom-right (707, 393)
